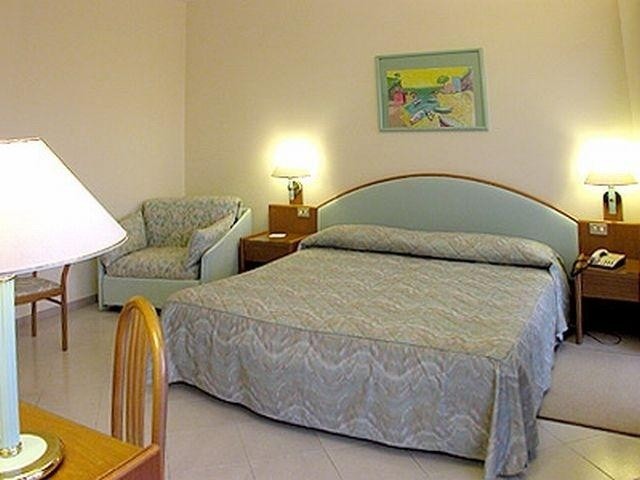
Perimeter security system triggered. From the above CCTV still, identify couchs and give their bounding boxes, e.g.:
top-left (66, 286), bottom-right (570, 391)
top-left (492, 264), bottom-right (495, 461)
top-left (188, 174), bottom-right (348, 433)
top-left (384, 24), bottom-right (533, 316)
top-left (98, 196), bottom-right (251, 311)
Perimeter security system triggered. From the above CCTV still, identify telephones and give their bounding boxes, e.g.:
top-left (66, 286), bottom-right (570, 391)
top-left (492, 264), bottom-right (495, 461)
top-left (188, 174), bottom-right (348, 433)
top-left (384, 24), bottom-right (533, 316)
top-left (571, 249), bottom-right (626, 277)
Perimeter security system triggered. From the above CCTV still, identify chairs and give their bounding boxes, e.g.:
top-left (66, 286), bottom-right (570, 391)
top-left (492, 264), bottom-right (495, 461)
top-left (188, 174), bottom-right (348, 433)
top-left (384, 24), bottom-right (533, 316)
top-left (14, 264), bottom-right (69, 352)
top-left (109, 295), bottom-right (169, 448)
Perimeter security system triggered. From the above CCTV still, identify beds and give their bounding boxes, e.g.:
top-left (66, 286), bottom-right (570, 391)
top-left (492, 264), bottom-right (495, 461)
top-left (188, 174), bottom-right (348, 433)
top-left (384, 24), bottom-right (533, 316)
top-left (138, 173), bottom-right (579, 480)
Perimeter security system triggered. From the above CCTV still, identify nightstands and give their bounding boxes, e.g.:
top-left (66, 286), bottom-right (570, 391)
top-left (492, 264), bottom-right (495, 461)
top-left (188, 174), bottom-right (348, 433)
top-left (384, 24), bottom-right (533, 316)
top-left (571, 219), bottom-right (640, 344)
top-left (237, 206), bottom-right (316, 273)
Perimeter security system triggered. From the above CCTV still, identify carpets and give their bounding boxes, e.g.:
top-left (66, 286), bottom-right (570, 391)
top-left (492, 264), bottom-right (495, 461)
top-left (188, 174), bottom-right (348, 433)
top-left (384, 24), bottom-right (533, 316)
top-left (536, 327), bottom-right (640, 437)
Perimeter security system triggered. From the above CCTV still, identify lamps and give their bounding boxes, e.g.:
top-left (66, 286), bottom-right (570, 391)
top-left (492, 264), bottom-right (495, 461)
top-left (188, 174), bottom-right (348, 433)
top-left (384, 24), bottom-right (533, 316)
top-left (269, 135), bottom-right (324, 206)
top-left (0, 137), bottom-right (129, 480)
top-left (583, 136), bottom-right (639, 222)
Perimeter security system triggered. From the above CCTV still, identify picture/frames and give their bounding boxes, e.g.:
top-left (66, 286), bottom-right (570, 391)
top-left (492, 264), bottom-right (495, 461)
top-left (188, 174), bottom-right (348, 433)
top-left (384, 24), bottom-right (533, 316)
top-left (374, 48), bottom-right (490, 133)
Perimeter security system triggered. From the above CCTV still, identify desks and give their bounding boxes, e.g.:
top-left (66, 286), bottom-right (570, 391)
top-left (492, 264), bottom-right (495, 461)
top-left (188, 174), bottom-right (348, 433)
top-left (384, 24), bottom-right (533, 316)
top-left (19, 400), bottom-right (159, 480)
